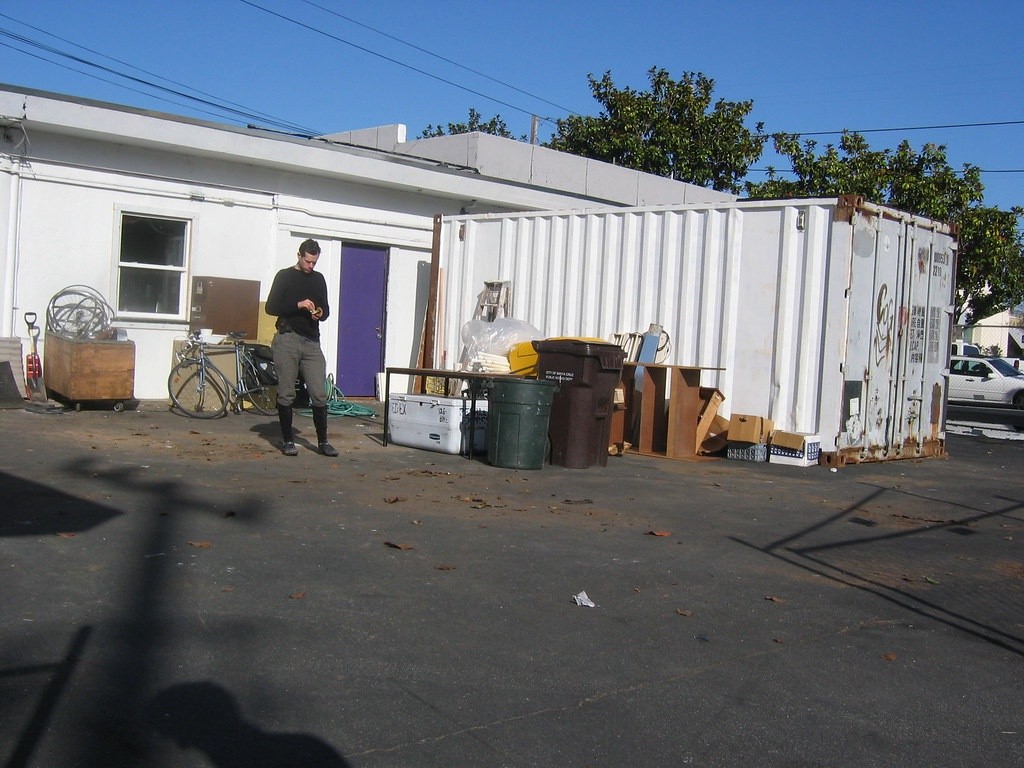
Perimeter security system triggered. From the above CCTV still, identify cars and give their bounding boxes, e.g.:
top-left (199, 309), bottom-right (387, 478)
top-left (952, 343), bottom-right (981, 356)
top-left (947, 354), bottom-right (1024, 410)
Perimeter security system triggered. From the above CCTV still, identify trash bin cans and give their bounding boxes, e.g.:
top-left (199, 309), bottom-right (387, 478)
top-left (530, 338), bottom-right (628, 469)
top-left (486, 376), bottom-right (556, 470)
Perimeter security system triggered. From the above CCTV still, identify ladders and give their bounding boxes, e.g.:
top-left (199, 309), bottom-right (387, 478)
top-left (450, 280), bottom-right (512, 398)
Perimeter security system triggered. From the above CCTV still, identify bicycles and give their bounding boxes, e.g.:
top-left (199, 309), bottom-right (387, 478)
top-left (167, 329), bottom-right (279, 419)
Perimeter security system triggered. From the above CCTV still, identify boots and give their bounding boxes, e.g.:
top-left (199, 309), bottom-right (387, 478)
top-left (311, 405), bottom-right (339, 456)
top-left (277, 402), bottom-right (298, 455)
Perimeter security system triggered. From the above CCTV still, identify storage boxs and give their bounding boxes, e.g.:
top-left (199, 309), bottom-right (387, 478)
top-left (387, 391), bottom-right (492, 455)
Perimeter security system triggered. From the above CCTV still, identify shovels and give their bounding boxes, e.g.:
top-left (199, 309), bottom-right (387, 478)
top-left (24, 311), bottom-right (48, 402)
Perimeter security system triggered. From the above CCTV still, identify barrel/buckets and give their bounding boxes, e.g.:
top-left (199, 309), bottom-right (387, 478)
top-left (484, 377), bottom-right (557, 470)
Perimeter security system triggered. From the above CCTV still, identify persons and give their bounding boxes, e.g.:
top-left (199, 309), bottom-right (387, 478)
top-left (264, 238), bottom-right (340, 457)
